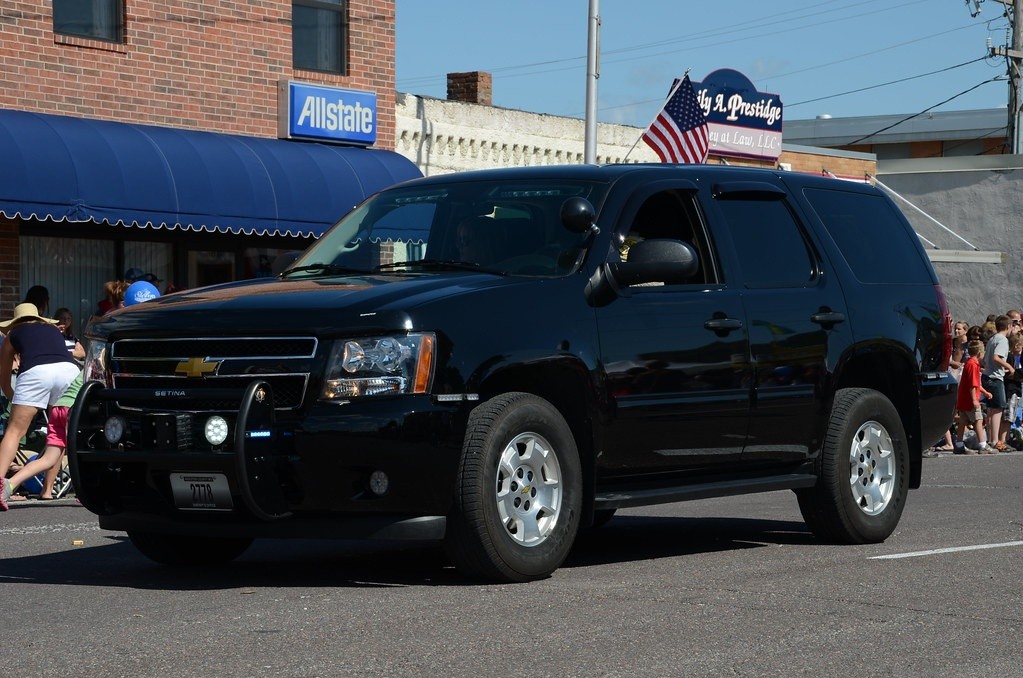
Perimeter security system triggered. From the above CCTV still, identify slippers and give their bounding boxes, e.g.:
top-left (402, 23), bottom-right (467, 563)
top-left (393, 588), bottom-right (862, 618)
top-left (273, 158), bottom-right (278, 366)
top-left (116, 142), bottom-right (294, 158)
top-left (38, 496), bottom-right (62, 500)
top-left (932, 446), bottom-right (954, 452)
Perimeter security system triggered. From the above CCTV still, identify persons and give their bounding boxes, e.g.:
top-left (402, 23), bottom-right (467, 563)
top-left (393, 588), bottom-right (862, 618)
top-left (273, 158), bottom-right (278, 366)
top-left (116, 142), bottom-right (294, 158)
top-left (0, 285), bottom-right (81, 500)
top-left (440, 218), bottom-right (498, 266)
top-left (53, 308), bottom-right (85, 361)
top-left (931, 310), bottom-right (1023, 454)
top-left (0, 368), bottom-right (84, 510)
top-left (97, 268), bottom-right (179, 317)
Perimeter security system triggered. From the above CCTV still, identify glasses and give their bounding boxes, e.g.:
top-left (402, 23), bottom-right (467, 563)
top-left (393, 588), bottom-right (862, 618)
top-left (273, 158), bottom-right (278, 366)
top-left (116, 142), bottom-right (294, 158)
top-left (455, 240), bottom-right (471, 248)
top-left (1012, 319), bottom-right (1022, 324)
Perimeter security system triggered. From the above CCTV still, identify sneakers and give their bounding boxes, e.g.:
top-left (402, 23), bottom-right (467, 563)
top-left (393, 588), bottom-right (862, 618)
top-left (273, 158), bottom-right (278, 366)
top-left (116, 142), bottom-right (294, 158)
top-left (953, 443), bottom-right (975, 455)
top-left (994, 440), bottom-right (1012, 453)
top-left (1016, 427), bottom-right (1023, 442)
top-left (978, 444), bottom-right (999, 455)
top-left (0, 477), bottom-right (14, 511)
top-left (1004, 443), bottom-right (1017, 452)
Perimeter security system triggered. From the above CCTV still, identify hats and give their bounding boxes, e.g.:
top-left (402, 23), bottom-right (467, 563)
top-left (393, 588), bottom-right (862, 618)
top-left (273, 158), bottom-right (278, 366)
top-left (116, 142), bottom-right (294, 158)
top-left (144, 273), bottom-right (165, 283)
top-left (0, 303), bottom-right (61, 335)
top-left (614, 201), bottom-right (663, 238)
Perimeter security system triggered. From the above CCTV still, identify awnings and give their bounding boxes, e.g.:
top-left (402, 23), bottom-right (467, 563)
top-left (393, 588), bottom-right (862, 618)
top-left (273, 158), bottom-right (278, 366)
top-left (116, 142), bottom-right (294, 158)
top-left (0, 109), bottom-right (436, 245)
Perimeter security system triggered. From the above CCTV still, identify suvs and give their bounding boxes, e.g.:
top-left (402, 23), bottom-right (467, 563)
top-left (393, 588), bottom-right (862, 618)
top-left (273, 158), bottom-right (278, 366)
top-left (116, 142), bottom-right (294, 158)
top-left (66, 163), bottom-right (958, 583)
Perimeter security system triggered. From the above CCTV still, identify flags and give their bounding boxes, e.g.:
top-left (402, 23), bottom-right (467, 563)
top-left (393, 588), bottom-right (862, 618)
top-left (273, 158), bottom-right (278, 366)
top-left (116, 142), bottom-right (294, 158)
top-left (642, 75), bottom-right (709, 164)
